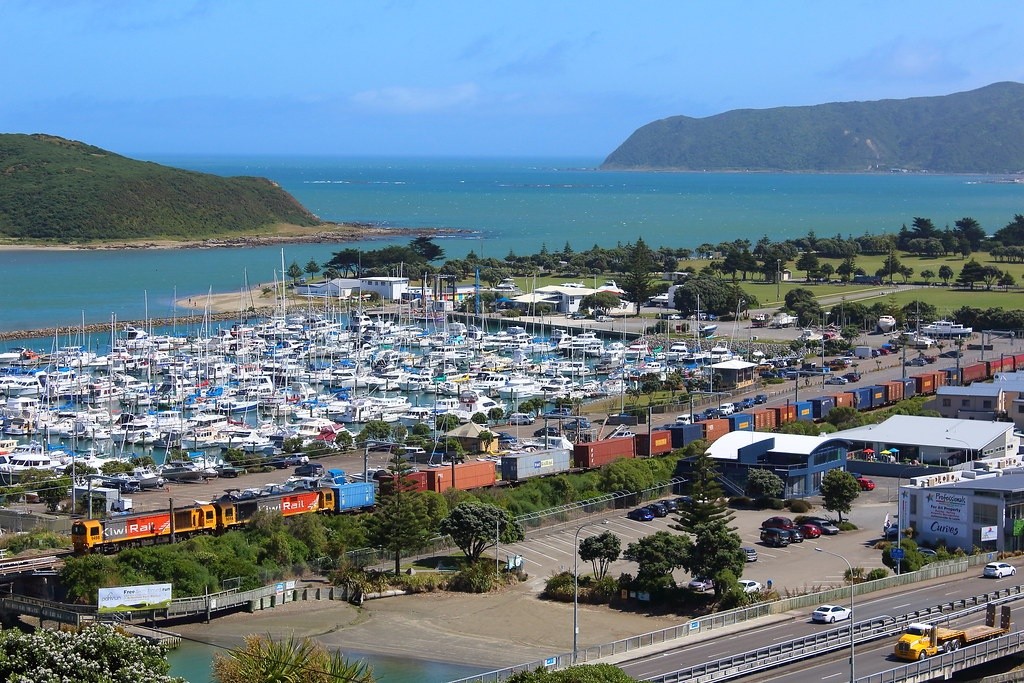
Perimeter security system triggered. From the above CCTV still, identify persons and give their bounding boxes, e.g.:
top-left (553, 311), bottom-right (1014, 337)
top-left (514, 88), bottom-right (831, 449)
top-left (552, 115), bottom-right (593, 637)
top-left (886, 519), bottom-right (891, 527)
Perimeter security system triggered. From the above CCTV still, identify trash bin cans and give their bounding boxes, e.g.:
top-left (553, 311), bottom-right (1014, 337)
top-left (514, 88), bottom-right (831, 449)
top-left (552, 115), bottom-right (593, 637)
top-left (318, 587), bottom-right (330, 600)
top-left (261, 595), bottom-right (272, 609)
top-left (305, 588), bottom-right (318, 601)
top-left (284, 591), bottom-right (293, 604)
top-left (766, 580), bottom-right (772, 589)
top-left (271, 593), bottom-right (284, 608)
top-left (294, 589), bottom-right (304, 601)
top-left (331, 586), bottom-right (347, 600)
top-left (247, 598), bottom-right (261, 613)
top-left (505, 554), bottom-right (523, 573)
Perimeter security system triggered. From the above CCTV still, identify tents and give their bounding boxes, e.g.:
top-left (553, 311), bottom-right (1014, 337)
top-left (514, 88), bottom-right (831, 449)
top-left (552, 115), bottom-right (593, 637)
top-left (864, 448), bottom-right (900, 463)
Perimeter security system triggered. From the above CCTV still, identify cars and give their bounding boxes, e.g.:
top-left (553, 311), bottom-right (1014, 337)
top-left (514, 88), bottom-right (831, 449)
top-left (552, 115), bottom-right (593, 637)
top-left (627, 496), bottom-right (699, 521)
top-left (688, 574), bottom-right (716, 593)
top-left (916, 546), bottom-right (938, 559)
top-left (884, 526), bottom-right (898, 541)
top-left (850, 473), bottom-right (862, 480)
top-left (729, 580), bottom-right (763, 596)
top-left (760, 514), bottom-right (840, 549)
top-left (812, 604), bottom-right (852, 624)
top-left (983, 561), bottom-right (1017, 578)
top-left (739, 546), bottom-right (758, 562)
top-left (856, 477), bottom-right (876, 491)
top-left (72, 310), bottom-right (973, 494)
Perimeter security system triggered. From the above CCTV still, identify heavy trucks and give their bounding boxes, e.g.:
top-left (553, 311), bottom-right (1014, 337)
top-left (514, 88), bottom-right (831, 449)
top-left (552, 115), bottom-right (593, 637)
top-left (894, 603), bottom-right (1012, 662)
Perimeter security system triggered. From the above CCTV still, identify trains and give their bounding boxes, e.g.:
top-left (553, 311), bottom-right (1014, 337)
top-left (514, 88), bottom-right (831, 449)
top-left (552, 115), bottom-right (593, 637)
top-left (71, 342), bottom-right (1024, 558)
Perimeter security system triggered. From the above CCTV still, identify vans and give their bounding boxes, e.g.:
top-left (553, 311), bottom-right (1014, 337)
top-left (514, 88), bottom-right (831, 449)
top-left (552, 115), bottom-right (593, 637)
top-left (882, 512), bottom-right (899, 531)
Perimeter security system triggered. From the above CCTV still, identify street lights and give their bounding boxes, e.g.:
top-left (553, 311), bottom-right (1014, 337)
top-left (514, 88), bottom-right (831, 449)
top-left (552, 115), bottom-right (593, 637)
top-left (815, 547), bottom-right (855, 683)
top-left (946, 436), bottom-right (973, 471)
top-left (572, 520), bottom-right (610, 661)
top-left (898, 465), bottom-right (929, 575)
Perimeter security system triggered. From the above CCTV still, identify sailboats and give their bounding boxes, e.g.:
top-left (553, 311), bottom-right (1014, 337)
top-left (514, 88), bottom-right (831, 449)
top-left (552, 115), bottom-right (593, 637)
top-left (1, 235), bottom-right (773, 540)
